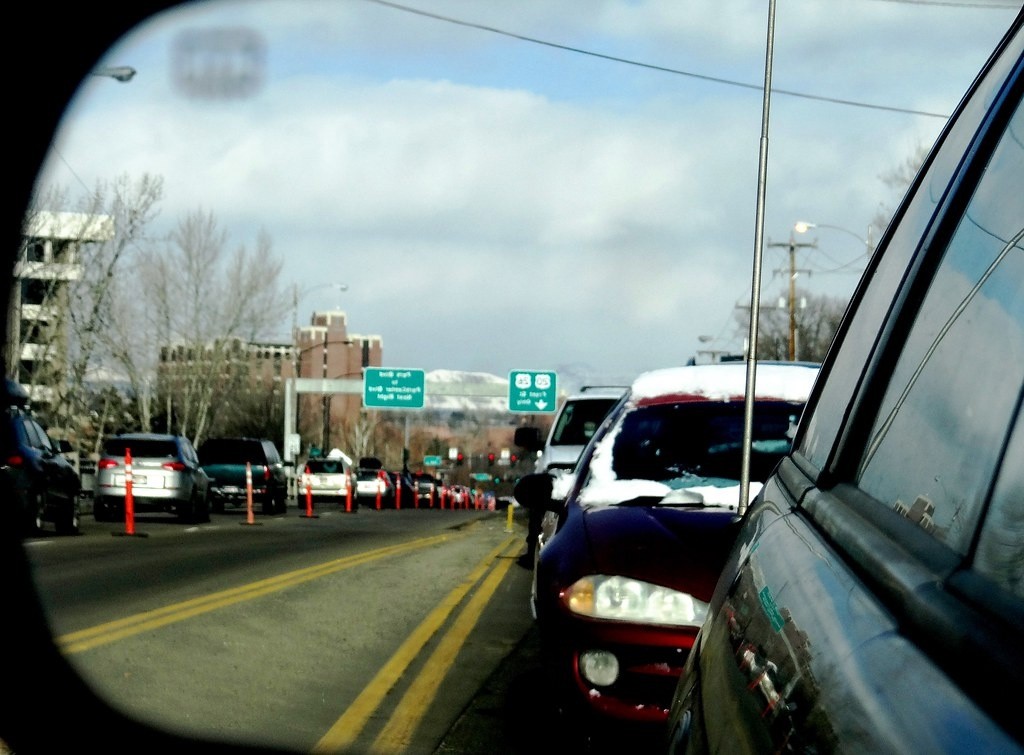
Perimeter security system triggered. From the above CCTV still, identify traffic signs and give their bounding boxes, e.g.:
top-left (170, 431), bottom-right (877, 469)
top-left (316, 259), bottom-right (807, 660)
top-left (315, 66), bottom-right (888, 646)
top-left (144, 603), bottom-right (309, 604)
top-left (508, 370), bottom-right (556, 412)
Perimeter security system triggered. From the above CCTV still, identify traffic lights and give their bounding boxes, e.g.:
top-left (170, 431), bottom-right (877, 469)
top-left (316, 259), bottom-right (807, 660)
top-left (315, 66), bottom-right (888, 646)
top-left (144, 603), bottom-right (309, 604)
top-left (457, 453), bottom-right (463, 466)
top-left (486, 452), bottom-right (495, 467)
top-left (510, 455), bottom-right (518, 469)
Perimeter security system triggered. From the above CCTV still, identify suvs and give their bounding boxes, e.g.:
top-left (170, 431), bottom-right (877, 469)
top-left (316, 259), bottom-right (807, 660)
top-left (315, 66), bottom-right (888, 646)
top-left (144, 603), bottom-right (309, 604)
top-left (297, 457), bottom-right (359, 512)
top-left (198, 437), bottom-right (289, 514)
top-left (94, 434), bottom-right (212, 522)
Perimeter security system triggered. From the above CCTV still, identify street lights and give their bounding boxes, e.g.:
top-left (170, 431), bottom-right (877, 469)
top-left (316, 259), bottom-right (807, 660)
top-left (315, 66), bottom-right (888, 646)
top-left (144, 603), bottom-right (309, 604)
top-left (327, 372), bottom-right (364, 454)
top-left (372, 416), bottom-right (406, 457)
top-left (296, 340), bottom-right (354, 469)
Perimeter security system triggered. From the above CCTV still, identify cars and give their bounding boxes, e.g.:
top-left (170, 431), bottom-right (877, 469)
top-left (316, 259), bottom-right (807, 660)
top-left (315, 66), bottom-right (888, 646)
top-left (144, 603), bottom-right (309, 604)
top-left (387, 470), bottom-right (415, 508)
top-left (350, 466), bottom-right (396, 509)
top-left (662, 5), bottom-right (1023, 755)
top-left (4, 378), bottom-right (81, 536)
top-left (514, 385), bottom-right (633, 558)
top-left (436, 480), bottom-right (498, 509)
top-left (512, 360), bottom-right (826, 738)
top-left (410, 472), bottom-right (439, 508)
